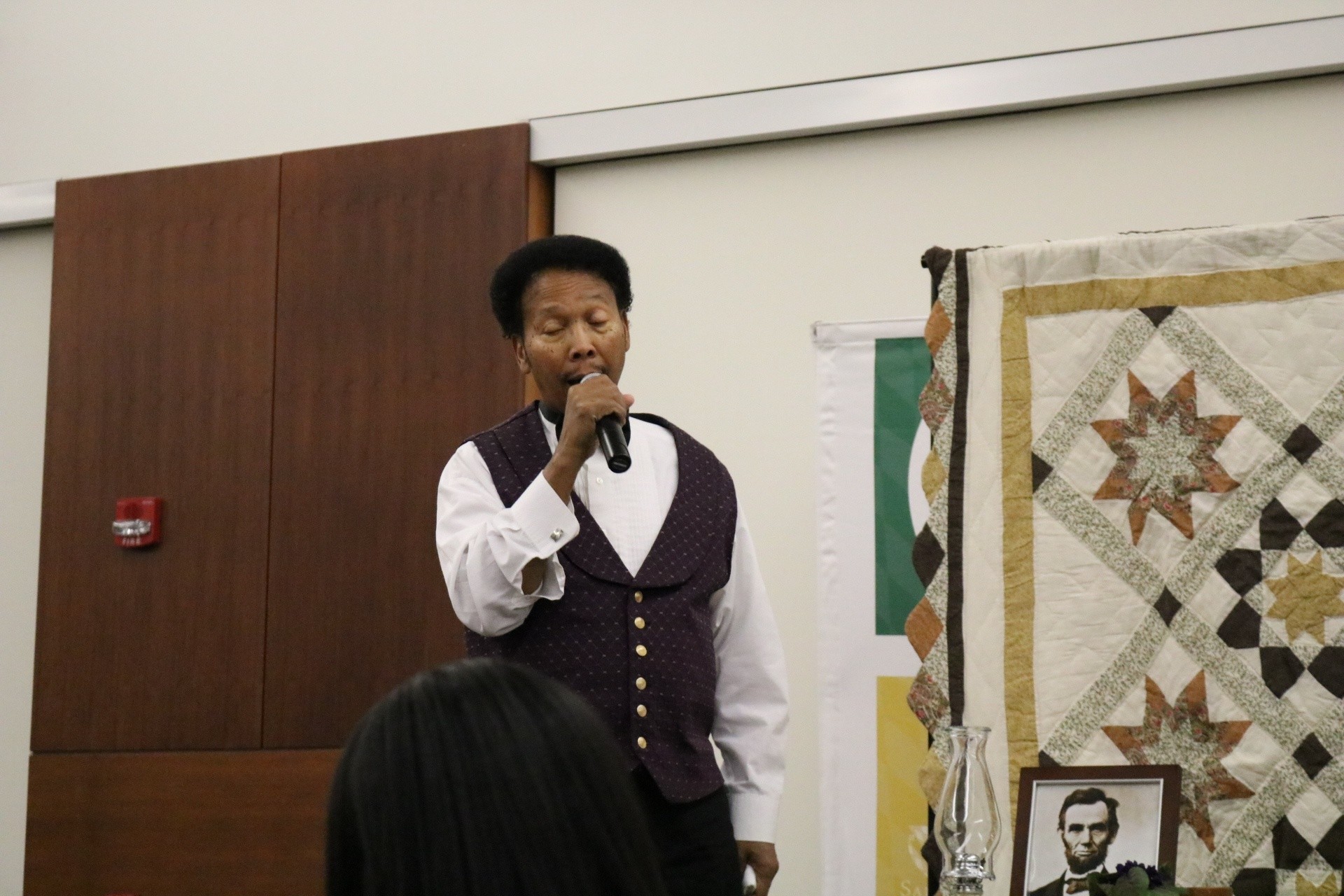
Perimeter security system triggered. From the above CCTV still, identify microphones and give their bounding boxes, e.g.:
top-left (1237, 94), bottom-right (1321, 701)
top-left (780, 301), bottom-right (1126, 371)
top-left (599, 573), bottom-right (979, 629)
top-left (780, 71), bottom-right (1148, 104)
top-left (580, 372), bottom-right (632, 474)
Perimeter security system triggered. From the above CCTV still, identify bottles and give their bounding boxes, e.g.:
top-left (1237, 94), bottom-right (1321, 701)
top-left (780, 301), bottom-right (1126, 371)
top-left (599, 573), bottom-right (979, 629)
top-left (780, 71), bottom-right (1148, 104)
top-left (932, 726), bottom-right (1001, 881)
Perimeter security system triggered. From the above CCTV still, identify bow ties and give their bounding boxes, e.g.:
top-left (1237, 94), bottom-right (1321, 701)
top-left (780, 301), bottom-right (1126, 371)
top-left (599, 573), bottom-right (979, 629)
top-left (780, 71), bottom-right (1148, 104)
top-left (1065, 876), bottom-right (1091, 894)
top-left (538, 400), bottom-right (631, 449)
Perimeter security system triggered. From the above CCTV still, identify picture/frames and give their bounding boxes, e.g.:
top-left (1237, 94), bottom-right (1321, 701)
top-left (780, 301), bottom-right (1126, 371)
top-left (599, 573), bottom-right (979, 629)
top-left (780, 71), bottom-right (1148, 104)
top-left (1009, 762), bottom-right (1188, 896)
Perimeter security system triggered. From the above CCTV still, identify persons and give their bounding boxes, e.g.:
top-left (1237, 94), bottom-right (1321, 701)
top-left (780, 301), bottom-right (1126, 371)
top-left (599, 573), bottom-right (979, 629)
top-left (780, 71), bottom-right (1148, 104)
top-left (325, 658), bottom-right (666, 896)
top-left (1027, 787), bottom-right (1120, 896)
top-left (435, 236), bottom-right (792, 896)
